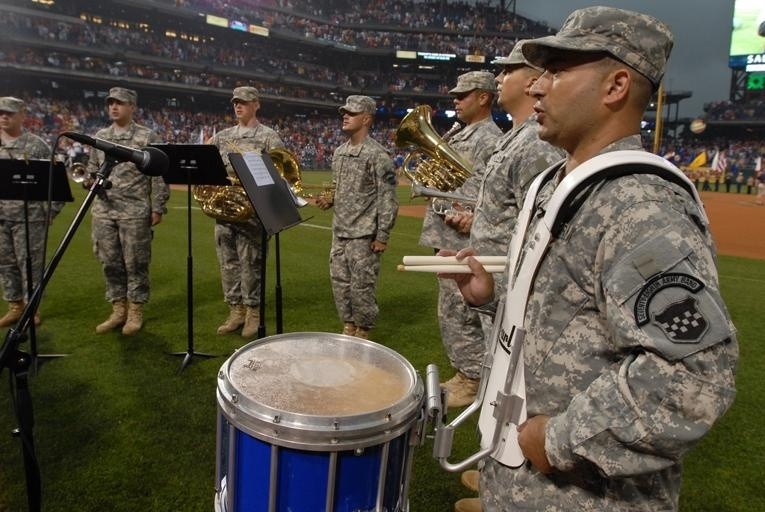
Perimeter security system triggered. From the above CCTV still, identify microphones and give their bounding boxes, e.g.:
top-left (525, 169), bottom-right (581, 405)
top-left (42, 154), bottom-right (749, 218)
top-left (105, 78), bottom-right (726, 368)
top-left (63, 131), bottom-right (170, 179)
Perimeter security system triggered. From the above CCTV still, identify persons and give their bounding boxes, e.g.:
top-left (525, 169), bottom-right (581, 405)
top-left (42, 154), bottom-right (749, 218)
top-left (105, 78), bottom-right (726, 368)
top-left (440, 34), bottom-right (569, 511)
top-left (643, 93), bottom-right (765, 195)
top-left (194, 82), bottom-right (289, 340)
top-left (0, 94), bottom-right (70, 330)
top-left (80, 85), bottom-right (171, 338)
top-left (415, 66), bottom-right (506, 409)
top-left (315, 93), bottom-right (400, 342)
top-left (1, 1), bottom-right (558, 171)
top-left (435, 3), bottom-right (743, 511)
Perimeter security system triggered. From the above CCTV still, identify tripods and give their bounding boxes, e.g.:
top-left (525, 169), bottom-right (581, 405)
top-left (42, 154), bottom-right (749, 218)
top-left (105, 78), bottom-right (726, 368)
top-left (9, 180), bottom-right (73, 376)
top-left (163, 166), bottom-right (219, 376)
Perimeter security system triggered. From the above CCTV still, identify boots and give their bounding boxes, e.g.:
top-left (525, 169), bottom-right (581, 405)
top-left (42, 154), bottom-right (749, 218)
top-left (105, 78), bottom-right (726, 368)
top-left (241, 304), bottom-right (261, 337)
top-left (439, 371), bottom-right (484, 512)
top-left (121, 303), bottom-right (143, 336)
top-left (0, 299), bottom-right (25, 326)
top-left (95, 298), bottom-right (127, 332)
top-left (352, 327), bottom-right (369, 339)
top-left (342, 321), bottom-right (355, 335)
top-left (25, 300), bottom-right (40, 324)
top-left (217, 304), bottom-right (244, 334)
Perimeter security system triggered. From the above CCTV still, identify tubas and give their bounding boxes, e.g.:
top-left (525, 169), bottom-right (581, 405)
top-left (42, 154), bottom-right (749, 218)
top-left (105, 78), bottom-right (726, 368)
top-left (394, 104), bottom-right (477, 193)
top-left (193, 148), bottom-right (302, 221)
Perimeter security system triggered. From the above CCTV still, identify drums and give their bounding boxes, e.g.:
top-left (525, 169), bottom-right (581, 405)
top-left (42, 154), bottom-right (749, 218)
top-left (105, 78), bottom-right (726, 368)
top-left (213, 331), bottom-right (427, 512)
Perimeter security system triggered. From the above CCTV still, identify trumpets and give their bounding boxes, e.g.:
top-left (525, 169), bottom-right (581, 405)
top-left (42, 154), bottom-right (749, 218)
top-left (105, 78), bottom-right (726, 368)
top-left (68, 163), bottom-right (93, 190)
top-left (409, 175), bottom-right (479, 216)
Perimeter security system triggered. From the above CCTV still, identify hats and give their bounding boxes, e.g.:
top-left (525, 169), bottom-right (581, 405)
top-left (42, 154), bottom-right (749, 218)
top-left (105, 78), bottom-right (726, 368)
top-left (0, 96), bottom-right (24, 113)
top-left (338, 94), bottom-right (377, 116)
top-left (230, 86), bottom-right (259, 104)
top-left (490, 35), bottom-right (543, 73)
top-left (522, 6), bottom-right (674, 94)
top-left (104, 87), bottom-right (137, 105)
top-left (448, 71), bottom-right (497, 95)
